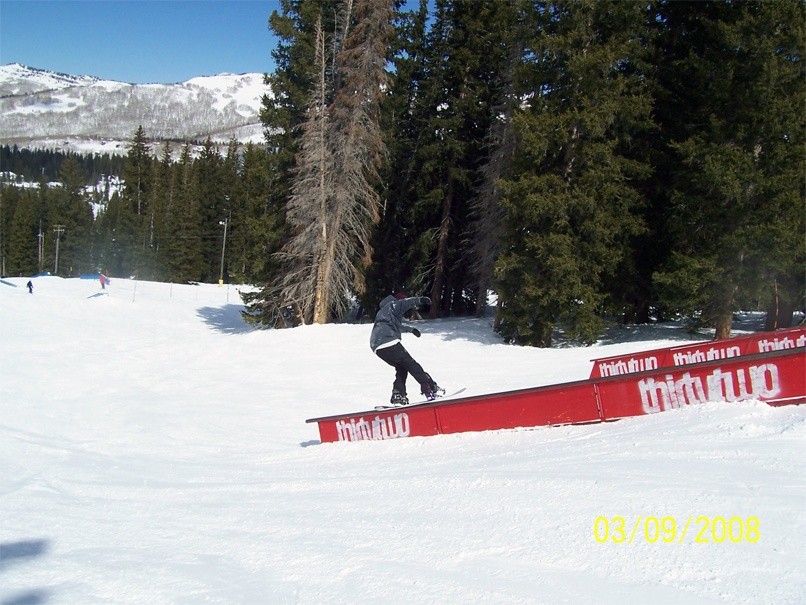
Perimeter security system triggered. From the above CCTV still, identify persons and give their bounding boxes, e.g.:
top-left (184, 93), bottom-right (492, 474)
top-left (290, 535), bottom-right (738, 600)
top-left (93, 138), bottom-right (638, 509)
top-left (26, 280), bottom-right (33, 293)
top-left (369, 292), bottom-right (446, 406)
top-left (97, 270), bottom-right (111, 289)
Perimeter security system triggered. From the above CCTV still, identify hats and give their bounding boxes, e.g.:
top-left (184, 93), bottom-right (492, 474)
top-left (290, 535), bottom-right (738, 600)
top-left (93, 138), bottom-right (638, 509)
top-left (395, 292), bottom-right (407, 300)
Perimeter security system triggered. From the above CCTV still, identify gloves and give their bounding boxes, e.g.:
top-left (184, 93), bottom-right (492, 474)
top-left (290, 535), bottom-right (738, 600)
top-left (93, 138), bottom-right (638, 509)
top-left (421, 297), bottom-right (432, 304)
top-left (412, 328), bottom-right (421, 337)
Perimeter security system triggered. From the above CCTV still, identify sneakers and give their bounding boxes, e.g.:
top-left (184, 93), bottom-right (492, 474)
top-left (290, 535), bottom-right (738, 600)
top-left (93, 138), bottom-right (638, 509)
top-left (391, 394), bottom-right (409, 405)
top-left (426, 386), bottom-right (445, 400)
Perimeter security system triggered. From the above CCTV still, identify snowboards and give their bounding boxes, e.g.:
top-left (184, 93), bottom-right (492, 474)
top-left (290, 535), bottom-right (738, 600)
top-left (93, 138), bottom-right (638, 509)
top-left (375, 388), bottom-right (466, 410)
